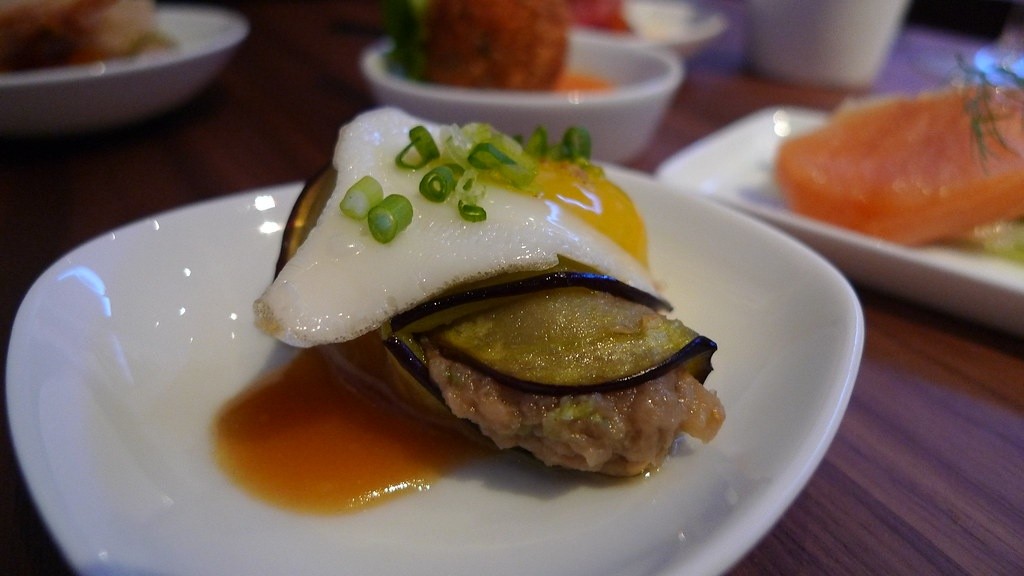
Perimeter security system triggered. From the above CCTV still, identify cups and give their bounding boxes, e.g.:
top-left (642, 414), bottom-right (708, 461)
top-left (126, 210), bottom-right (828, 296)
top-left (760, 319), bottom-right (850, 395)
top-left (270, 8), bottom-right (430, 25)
top-left (747, 0), bottom-right (910, 88)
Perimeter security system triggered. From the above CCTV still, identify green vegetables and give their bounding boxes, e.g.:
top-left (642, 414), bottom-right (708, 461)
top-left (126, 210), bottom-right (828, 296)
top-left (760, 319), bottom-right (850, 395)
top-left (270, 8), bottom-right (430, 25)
top-left (949, 52), bottom-right (1024, 172)
top-left (377, 0), bottom-right (439, 81)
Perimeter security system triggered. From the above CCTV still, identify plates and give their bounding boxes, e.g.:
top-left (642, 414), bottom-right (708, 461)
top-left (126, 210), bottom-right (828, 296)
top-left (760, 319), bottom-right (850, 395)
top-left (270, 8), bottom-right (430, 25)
top-left (613, 2), bottom-right (728, 59)
top-left (653, 101), bottom-right (1024, 349)
top-left (6, 162), bottom-right (865, 576)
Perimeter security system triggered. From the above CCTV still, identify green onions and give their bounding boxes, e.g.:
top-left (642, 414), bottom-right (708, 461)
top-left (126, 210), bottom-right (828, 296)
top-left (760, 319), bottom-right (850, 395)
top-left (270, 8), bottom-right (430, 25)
top-left (340, 121), bottom-right (593, 243)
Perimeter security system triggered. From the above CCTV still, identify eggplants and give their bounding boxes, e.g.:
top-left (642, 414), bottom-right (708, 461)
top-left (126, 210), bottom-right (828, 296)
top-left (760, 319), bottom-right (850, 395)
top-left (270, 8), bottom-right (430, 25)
top-left (281, 163), bottom-right (718, 464)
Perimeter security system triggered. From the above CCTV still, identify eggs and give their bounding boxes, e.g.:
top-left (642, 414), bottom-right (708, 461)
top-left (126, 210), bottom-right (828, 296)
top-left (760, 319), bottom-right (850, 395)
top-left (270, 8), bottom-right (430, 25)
top-left (509, 156), bottom-right (651, 271)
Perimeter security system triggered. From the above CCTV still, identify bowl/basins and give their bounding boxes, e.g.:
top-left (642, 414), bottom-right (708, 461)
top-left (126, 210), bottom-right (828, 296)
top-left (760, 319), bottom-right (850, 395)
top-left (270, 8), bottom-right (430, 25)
top-left (1, 8), bottom-right (249, 141)
top-left (358, 33), bottom-right (684, 167)
